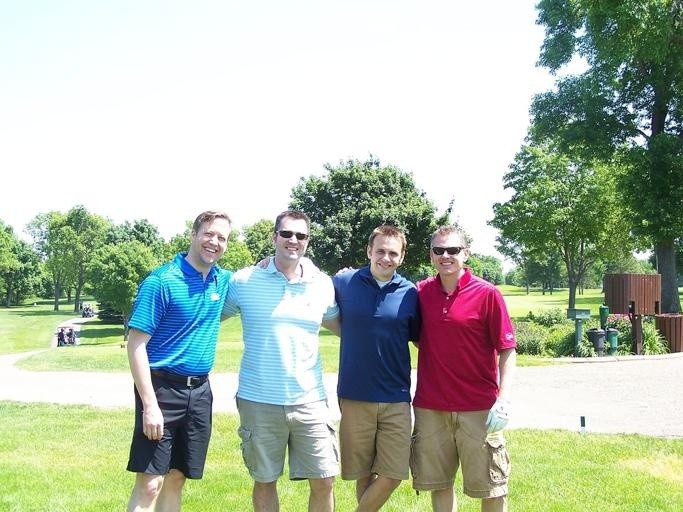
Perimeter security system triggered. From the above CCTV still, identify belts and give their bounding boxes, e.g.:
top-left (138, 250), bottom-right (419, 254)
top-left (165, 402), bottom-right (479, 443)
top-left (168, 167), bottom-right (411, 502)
top-left (151, 369), bottom-right (209, 387)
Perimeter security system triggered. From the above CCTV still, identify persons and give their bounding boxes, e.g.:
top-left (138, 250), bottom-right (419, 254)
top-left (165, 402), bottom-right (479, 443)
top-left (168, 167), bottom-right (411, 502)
top-left (252, 223), bottom-right (421, 512)
top-left (86, 308), bottom-right (91, 317)
top-left (219, 207), bottom-right (343, 512)
top-left (67, 329), bottom-right (76, 344)
top-left (333, 226), bottom-right (518, 512)
top-left (122, 209), bottom-right (236, 512)
top-left (58, 328), bottom-right (66, 344)
top-left (82, 308), bottom-right (86, 317)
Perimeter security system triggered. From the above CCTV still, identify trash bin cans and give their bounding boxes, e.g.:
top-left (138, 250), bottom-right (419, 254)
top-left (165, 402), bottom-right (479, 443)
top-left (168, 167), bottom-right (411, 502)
top-left (587, 328), bottom-right (606, 357)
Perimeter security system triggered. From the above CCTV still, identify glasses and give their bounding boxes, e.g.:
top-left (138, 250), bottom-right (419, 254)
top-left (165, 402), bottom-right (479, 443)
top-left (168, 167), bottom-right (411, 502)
top-left (431, 246), bottom-right (467, 255)
top-left (276, 230), bottom-right (309, 242)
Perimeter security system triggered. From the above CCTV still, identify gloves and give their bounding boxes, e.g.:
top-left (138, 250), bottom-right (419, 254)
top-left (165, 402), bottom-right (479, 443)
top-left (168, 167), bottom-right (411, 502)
top-left (485, 398), bottom-right (509, 434)
